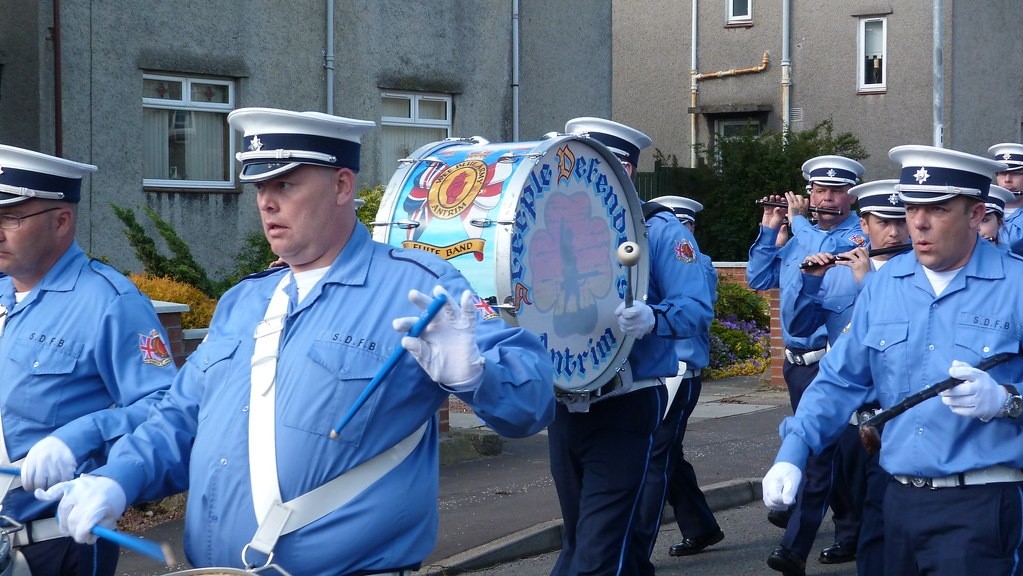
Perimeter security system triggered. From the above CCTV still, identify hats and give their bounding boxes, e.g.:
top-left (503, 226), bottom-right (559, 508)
top-left (801, 142), bottom-right (1022, 218)
top-left (227, 107), bottom-right (376, 183)
top-left (564, 116), bottom-right (653, 166)
top-left (646, 196), bottom-right (703, 225)
top-left (0, 139), bottom-right (99, 208)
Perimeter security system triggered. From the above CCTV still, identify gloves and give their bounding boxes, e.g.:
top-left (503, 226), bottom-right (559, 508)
top-left (393, 285), bottom-right (483, 384)
top-left (939, 360), bottom-right (1004, 422)
top-left (762, 462), bottom-right (802, 512)
top-left (616, 300), bottom-right (656, 339)
top-left (22, 434), bottom-right (77, 492)
top-left (33, 473), bottom-right (125, 545)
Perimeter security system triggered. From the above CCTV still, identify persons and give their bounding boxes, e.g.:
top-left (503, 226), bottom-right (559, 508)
top-left (34, 107), bottom-right (557, 576)
top-left (746, 142), bottom-right (1023, 576)
top-left (550, 115), bottom-right (715, 576)
top-left (0, 141), bottom-right (181, 576)
top-left (647, 196), bottom-right (725, 556)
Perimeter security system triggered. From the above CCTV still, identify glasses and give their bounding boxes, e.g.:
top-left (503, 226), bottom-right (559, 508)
top-left (0, 206), bottom-right (63, 230)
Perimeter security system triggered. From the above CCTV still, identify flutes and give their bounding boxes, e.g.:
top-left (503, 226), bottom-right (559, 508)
top-left (799, 243), bottom-right (913, 269)
top-left (985, 237), bottom-right (995, 242)
top-left (754, 199), bottom-right (844, 216)
top-left (1011, 190), bottom-right (1023, 196)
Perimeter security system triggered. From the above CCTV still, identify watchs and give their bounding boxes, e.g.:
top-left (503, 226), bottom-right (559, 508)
top-left (1001, 385), bottom-right (1023, 419)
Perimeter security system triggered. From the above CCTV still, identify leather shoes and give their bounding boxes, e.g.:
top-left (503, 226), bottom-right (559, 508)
top-left (768, 548), bottom-right (808, 575)
top-left (819, 539), bottom-right (857, 563)
top-left (669, 520), bottom-right (725, 555)
top-left (767, 508), bottom-right (789, 529)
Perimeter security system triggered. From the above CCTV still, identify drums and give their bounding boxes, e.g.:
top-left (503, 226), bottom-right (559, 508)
top-left (160, 564), bottom-right (261, 576)
top-left (371, 131), bottom-right (652, 399)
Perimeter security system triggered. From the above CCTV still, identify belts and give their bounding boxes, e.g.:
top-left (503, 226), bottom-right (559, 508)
top-left (784, 348), bottom-right (826, 365)
top-left (1, 516), bottom-right (68, 546)
top-left (893, 464), bottom-right (1023, 489)
top-left (850, 408), bottom-right (883, 426)
top-left (624, 378), bottom-right (667, 394)
top-left (683, 369), bottom-right (702, 378)
